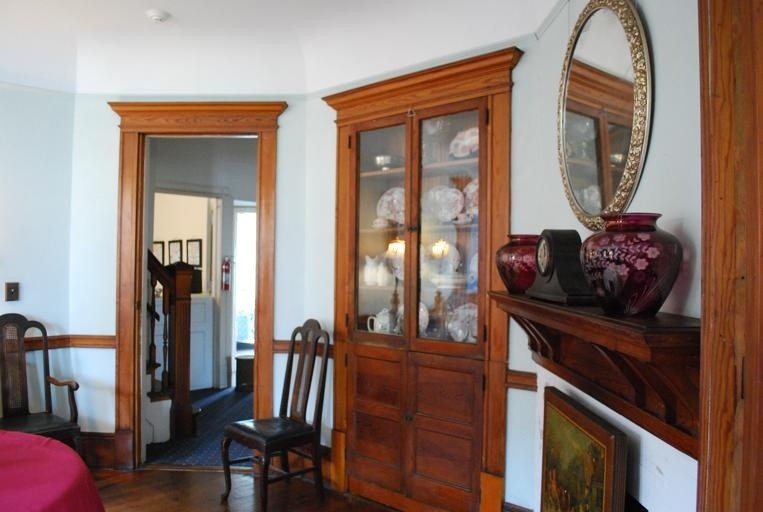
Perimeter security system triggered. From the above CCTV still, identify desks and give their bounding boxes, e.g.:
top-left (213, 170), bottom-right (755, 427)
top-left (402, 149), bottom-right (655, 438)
top-left (0, 429), bottom-right (105, 512)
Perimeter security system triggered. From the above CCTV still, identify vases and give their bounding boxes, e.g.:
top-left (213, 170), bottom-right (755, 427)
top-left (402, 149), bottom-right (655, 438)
top-left (496, 234), bottom-right (538, 293)
top-left (580, 211), bottom-right (684, 318)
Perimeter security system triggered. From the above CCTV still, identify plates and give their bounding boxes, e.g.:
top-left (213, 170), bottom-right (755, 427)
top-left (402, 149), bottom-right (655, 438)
top-left (447, 302), bottom-right (477, 344)
top-left (374, 128), bottom-right (479, 289)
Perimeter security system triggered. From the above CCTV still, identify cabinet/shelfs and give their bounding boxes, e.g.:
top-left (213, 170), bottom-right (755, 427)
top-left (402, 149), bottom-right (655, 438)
top-left (321, 47), bottom-right (524, 512)
top-left (565, 58), bottom-right (633, 216)
top-left (155, 296), bottom-right (213, 391)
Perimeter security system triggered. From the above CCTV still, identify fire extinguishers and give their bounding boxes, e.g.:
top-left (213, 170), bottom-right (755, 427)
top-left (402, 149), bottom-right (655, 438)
top-left (221, 257), bottom-right (234, 291)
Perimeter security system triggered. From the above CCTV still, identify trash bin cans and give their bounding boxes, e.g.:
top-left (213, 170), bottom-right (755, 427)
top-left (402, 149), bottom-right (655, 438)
top-left (235, 356), bottom-right (254, 392)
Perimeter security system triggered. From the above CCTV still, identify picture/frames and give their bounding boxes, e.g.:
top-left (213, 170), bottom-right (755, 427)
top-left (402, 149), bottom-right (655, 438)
top-left (169, 240), bottom-right (182, 265)
top-left (540, 386), bottom-right (628, 512)
top-left (153, 241), bottom-right (164, 265)
top-left (187, 239), bottom-right (202, 266)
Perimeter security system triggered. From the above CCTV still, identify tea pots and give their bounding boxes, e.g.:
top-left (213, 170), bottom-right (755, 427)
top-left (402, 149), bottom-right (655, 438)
top-left (367, 307), bottom-right (404, 335)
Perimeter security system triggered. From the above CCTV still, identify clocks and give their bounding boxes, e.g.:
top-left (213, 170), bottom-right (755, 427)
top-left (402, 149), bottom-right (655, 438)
top-left (522, 230), bottom-right (595, 306)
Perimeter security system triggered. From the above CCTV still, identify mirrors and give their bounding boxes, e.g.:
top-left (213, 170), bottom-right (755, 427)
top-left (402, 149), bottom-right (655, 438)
top-left (556, 0), bottom-right (652, 230)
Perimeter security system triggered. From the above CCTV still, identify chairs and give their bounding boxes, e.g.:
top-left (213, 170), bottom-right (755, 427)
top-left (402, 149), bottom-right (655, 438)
top-left (0, 314), bottom-right (84, 456)
top-left (221, 319), bottom-right (330, 512)
